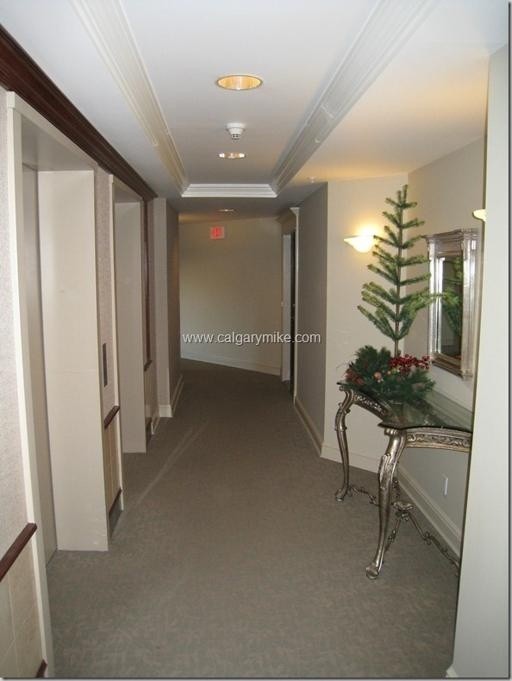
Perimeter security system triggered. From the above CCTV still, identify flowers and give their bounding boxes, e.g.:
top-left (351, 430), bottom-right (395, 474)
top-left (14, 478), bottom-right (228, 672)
top-left (344, 343), bottom-right (434, 404)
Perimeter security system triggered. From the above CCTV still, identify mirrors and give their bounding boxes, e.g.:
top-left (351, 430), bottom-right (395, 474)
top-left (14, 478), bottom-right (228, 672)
top-left (422, 226), bottom-right (480, 379)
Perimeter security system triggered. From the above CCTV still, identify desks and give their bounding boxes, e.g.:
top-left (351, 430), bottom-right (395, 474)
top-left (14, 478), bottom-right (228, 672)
top-left (332, 379), bottom-right (472, 580)
